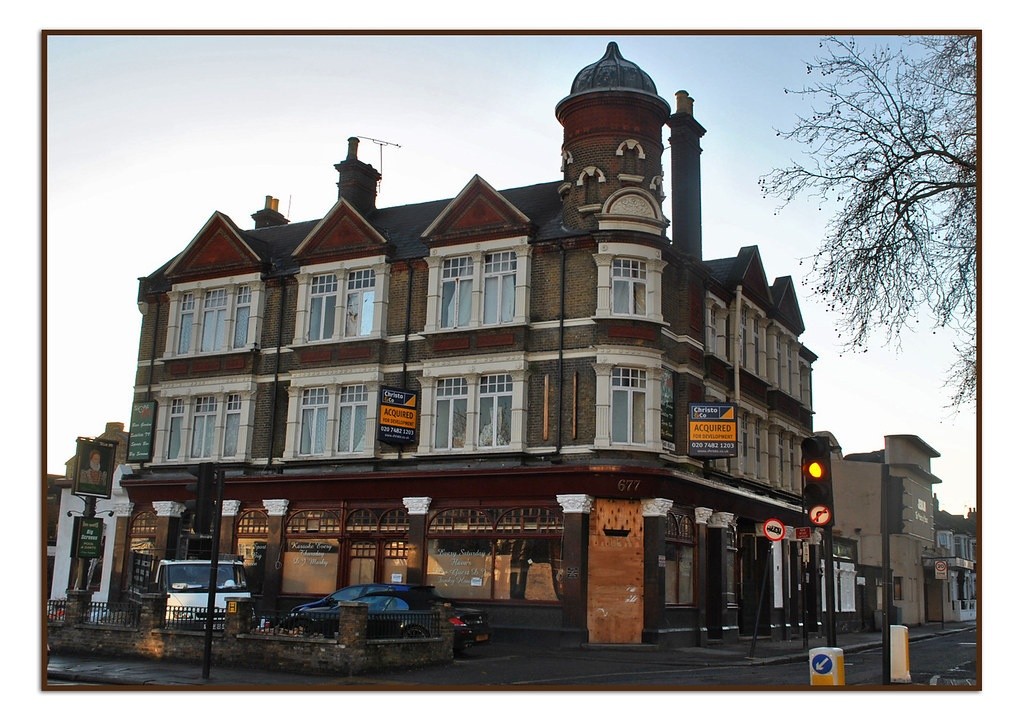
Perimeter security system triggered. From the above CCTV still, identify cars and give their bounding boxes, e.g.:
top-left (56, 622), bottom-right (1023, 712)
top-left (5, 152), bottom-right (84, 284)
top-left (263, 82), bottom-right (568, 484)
top-left (276, 590), bottom-right (491, 660)
top-left (289, 582), bottom-right (436, 616)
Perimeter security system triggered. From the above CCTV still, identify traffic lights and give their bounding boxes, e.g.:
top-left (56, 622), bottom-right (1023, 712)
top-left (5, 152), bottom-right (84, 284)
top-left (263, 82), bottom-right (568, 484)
top-left (801, 436), bottom-right (835, 528)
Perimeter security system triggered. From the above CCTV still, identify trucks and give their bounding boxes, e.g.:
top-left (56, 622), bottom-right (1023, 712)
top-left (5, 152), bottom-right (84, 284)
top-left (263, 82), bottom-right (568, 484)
top-left (130, 560), bottom-right (256, 631)
